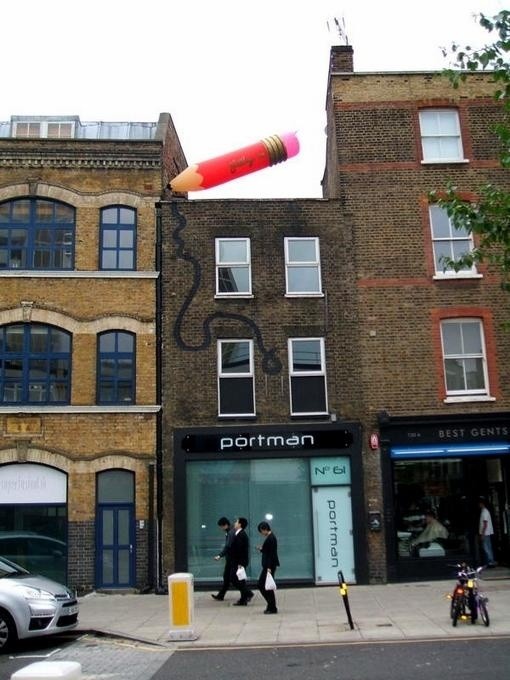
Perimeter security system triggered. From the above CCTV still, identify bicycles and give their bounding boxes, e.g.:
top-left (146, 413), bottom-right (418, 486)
top-left (447, 563), bottom-right (497, 627)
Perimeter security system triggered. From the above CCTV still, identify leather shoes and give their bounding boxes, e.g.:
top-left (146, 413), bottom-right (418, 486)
top-left (247, 593), bottom-right (253, 601)
top-left (233, 601), bottom-right (247, 606)
top-left (212, 595), bottom-right (224, 600)
top-left (264, 610), bottom-right (277, 614)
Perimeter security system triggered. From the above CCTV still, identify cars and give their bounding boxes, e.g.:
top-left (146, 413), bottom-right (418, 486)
top-left (0, 554), bottom-right (80, 649)
top-left (0, 532), bottom-right (67, 581)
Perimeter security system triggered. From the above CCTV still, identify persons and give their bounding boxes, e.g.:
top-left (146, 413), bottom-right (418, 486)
top-left (211, 516), bottom-right (254, 602)
top-left (410, 511), bottom-right (449, 552)
top-left (228, 516), bottom-right (250, 607)
top-left (477, 500), bottom-right (498, 569)
top-left (253, 521), bottom-right (280, 615)
